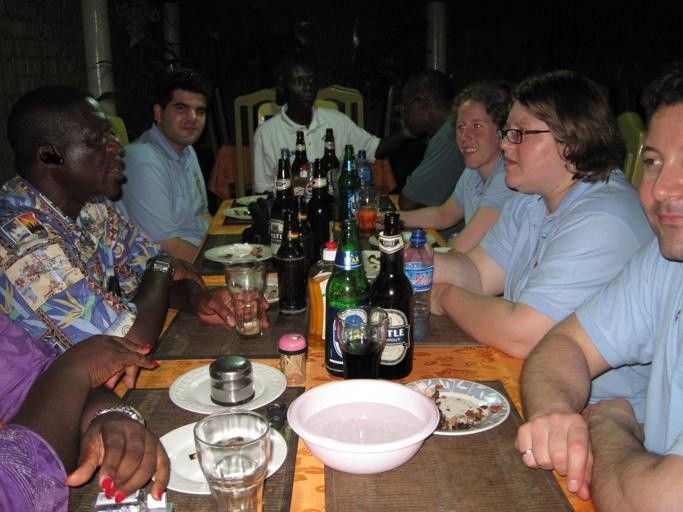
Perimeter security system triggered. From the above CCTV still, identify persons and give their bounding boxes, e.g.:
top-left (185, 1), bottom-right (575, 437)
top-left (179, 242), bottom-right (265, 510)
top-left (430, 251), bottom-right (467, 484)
top-left (250, 64), bottom-right (414, 196)
top-left (513, 72), bottom-right (683, 512)
top-left (405, 66), bottom-right (659, 425)
top-left (107, 77), bottom-right (213, 263)
top-left (0, 311), bottom-right (169, 512)
top-left (0, 86), bottom-right (269, 355)
top-left (372, 84), bottom-right (518, 251)
top-left (398, 70), bottom-right (465, 211)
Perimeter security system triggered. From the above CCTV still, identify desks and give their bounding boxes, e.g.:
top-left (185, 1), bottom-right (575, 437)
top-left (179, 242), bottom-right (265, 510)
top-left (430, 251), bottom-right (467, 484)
top-left (210, 145), bottom-right (395, 200)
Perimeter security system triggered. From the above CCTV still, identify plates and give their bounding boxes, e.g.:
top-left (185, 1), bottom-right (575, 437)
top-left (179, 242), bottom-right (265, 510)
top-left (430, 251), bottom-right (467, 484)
top-left (164, 359), bottom-right (286, 414)
top-left (149, 417), bottom-right (287, 495)
top-left (404, 375), bottom-right (512, 434)
top-left (203, 241), bottom-right (274, 262)
top-left (224, 193), bottom-right (270, 220)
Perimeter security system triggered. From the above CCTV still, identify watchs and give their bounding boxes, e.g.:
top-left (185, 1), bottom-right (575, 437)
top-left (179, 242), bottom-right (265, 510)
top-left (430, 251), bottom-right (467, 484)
top-left (91, 403), bottom-right (148, 428)
top-left (147, 259), bottom-right (174, 278)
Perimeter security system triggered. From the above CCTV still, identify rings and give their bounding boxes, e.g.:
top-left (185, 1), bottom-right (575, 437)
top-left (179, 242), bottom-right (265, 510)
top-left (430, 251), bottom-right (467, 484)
top-left (521, 449), bottom-right (531, 456)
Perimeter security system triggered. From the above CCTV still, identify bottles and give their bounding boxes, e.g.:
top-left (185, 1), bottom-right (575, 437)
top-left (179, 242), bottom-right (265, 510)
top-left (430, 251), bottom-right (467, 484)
top-left (336, 307), bottom-right (390, 378)
top-left (404, 229), bottom-right (434, 343)
top-left (281, 147), bottom-right (292, 177)
top-left (275, 209), bottom-right (309, 314)
top-left (319, 128), bottom-right (340, 201)
top-left (278, 334), bottom-right (308, 384)
top-left (368, 213), bottom-right (415, 379)
top-left (356, 149), bottom-right (375, 203)
top-left (272, 159), bottom-right (295, 260)
top-left (337, 144), bottom-right (363, 220)
top-left (306, 157), bottom-right (336, 276)
top-left (91, 489), bottom-right (167, 511)
top-left (323, 217), bottom-right (371, 377)
top-left (291, 131), bottom-right (309, 203)
top-left (209, 353), bottom-right (256, 405)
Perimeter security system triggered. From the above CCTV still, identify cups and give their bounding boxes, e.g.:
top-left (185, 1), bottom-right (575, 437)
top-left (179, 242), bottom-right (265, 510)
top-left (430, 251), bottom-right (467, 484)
top-left (195, 409), bottom-right (266, 512)
top-left (225, 258), bottom-right (267, 339)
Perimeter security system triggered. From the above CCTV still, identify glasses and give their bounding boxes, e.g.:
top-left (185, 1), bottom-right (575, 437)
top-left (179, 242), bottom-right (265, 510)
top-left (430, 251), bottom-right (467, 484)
top-left (394, 96), bottom-right (427, 112)
top-left (496, 129), bottom-right (552, 144)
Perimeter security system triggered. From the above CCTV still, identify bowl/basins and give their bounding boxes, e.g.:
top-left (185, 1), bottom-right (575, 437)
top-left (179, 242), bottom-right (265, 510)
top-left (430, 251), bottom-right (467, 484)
top-left (288, 379), bottom-right (441, 476)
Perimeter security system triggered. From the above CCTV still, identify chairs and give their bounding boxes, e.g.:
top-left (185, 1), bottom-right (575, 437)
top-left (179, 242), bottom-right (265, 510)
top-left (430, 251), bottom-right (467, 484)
top-left (616, 110), bottom-right (649, 191)
top-left (107, 112), bottom-right (129, 149)
top-left (209, 79), bottom-right (398, 200)
